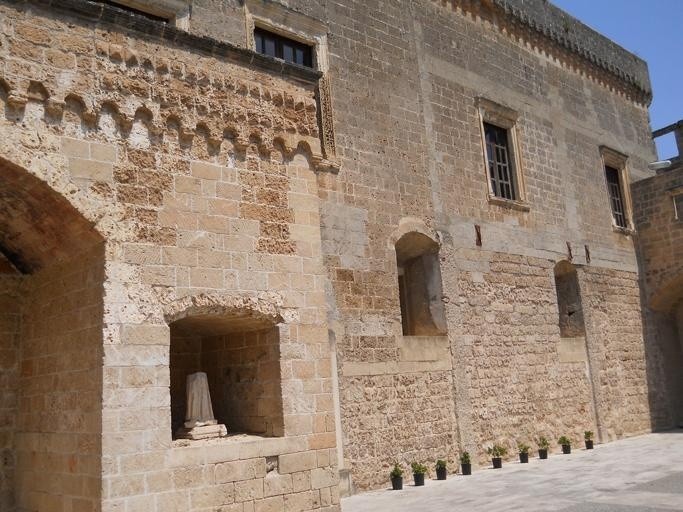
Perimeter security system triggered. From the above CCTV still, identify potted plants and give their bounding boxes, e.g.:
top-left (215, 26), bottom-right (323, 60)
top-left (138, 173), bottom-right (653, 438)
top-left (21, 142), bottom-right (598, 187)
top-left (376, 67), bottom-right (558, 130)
top-left (584, 431), bottom-right (594, 449)
top-left (488, 446), bottom-right (508, 468)
top-left (460, 452), bottom-right (471, 475)
top-left (558, 436), bottom-right (573, 454)
top-left (517, 442), bottom-right (531, 463)
top-left (390, 463), bottom-right (404, 490)
top-left (538, 436), bottom-right (550, 459)
top-left (411, 461), bottom-right (427, 486)
top-left (435, 460), bottom-right (447, 480)
